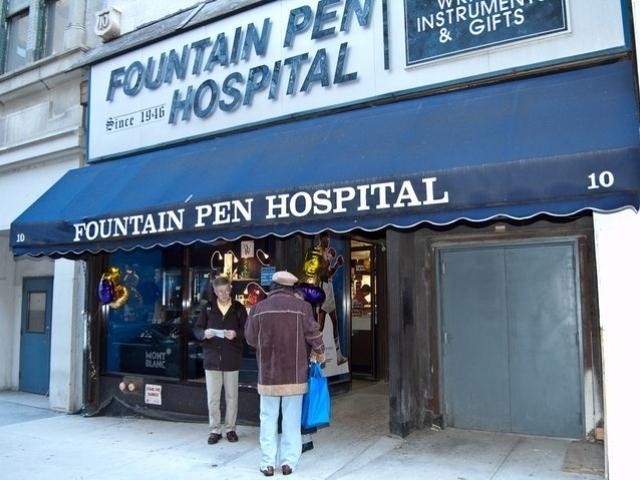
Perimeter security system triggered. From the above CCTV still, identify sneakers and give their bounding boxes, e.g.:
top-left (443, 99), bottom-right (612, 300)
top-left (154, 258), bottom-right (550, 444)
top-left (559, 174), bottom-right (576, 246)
top-left (302, 441), bottom-right (313, 453)
top-left (337, 355), bottom-right (349, 365)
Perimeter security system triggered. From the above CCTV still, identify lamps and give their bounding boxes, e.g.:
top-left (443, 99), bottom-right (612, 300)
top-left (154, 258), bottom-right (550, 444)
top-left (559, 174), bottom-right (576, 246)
top-left (209, 250), bottom-right (224, 271)
top-left (243, 280), bottom-right (268, 298)
top-left (255, 249), bottom-right (270, 266)
top-left (226, 249), bottom-right (239, 265)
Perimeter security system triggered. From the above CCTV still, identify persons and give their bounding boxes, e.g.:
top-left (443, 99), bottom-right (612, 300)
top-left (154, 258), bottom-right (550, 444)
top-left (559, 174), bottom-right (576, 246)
top-left (244, 270), bottom-right (325, 476)
top-left (193, 278), bottom-right (248, 443)
top-left (352, 285), bottom-right (370, 305)
top-left (308, 247), bottom-right (349, 368)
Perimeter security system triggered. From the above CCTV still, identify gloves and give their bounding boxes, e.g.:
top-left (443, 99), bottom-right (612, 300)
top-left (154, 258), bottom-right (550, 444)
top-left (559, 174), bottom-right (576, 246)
top-left (327, 247), bottom-right (336, 258)
top-left (336, 255), bottom-right (344, 265)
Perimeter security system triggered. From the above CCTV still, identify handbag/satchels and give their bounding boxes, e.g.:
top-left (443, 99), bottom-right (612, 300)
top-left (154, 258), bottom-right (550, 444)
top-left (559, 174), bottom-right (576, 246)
top-left (302, 362), bottom-right (332, 429)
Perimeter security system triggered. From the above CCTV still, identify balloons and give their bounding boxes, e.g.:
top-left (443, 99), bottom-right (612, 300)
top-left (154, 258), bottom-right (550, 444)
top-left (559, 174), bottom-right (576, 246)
top-left (97, 266), bottom-right (129, 309)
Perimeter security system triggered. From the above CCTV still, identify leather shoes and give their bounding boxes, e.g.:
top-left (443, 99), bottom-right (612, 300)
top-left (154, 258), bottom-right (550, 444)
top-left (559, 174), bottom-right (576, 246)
top-left (207, 432), bottom-right (222, 444)
top-left (226, 431), bottom-right (238, 442)
top-left (260, 464), bottom-right (274, 476)
top-left (282, 464), bottom-right (292, 475)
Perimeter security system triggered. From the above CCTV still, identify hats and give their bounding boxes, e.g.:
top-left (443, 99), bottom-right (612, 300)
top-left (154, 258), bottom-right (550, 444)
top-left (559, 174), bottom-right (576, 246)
top-left (272, 270), bottom-right (298, 286)
